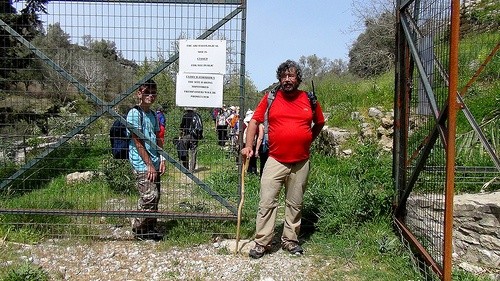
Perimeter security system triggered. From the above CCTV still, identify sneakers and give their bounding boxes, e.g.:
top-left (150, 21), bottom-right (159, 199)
top-left (249, 241), bottom-right (273, 259)
top-left (281, 239), bottom-right (303, 253)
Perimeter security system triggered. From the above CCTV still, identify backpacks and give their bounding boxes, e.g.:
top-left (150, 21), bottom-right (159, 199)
top-left (110, 105), bottom-right (157, 160)
top-left (189, 113), bottom-right (203, 136)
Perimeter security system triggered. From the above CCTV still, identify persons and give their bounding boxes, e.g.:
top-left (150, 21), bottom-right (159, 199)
top-left (155, 105), bottom-right (165, 144)
top-left (125, 79), bottom-right (165, 241)
top-left (178, 107), bottom-right (199, 173)
top-left (240, 59), bottom-right (325, 258)
top-left (210, 104), bottom-right (269, 177)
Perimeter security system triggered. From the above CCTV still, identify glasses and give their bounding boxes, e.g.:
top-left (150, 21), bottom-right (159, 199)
top-left (142, 90), bottom-right (156, 96)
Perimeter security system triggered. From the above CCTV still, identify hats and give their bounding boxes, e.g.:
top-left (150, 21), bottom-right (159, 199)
top-left (187, 107), bottom-right (194, 110)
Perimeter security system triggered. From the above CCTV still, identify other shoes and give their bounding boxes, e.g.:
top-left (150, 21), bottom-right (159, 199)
top-left (132, 226), bottom-right (164, 240)
